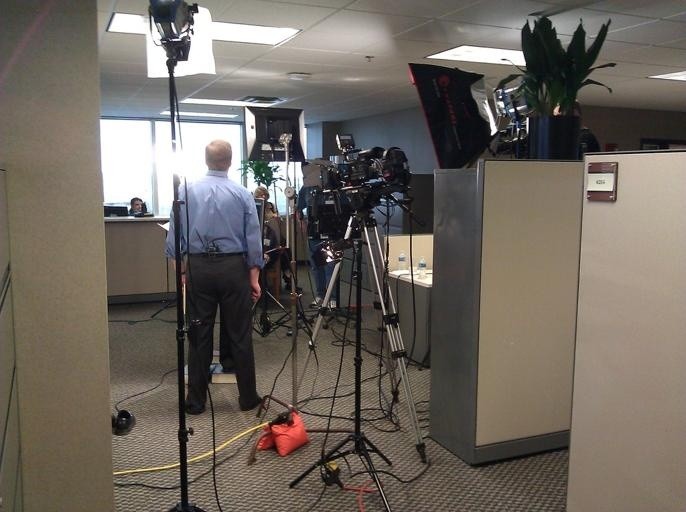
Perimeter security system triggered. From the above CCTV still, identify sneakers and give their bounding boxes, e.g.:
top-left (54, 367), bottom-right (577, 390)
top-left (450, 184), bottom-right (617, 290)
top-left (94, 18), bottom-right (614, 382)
top-left (282, 273), bottom-right (304, 291)
top-left (310, 297), bottom-right (338, 311)
top-left (112, 409), bottom-right (136, 436)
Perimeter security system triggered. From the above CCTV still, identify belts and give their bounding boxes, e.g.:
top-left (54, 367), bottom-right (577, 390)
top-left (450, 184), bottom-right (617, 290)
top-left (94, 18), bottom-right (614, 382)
top-left (190, 251), bottom-right (243, 258)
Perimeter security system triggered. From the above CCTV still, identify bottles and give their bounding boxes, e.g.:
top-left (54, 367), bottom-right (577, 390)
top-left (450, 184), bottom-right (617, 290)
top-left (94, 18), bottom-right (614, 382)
top-left (418, 256), bottom-right (426, 279)
top-left (398, 250), bottom-right (407, 273)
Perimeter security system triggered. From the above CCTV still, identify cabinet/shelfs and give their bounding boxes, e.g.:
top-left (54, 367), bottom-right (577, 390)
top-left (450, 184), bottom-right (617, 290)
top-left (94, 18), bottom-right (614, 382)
top-left (338, 173), bottom-right (434, 294)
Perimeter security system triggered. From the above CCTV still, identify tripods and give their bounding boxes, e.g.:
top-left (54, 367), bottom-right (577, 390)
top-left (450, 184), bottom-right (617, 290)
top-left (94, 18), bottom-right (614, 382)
top-left (151, 228), bottom-right (358, 335)
top-left (296, 205), bottom-right (428, 465)
top-left (289, 219), bottom-right (392, 511)
top-left (247, 181), bottom-right (354, 464)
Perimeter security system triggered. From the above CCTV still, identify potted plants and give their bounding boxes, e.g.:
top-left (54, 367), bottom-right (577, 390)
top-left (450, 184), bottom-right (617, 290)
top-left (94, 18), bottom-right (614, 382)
top-left (493, 15), bottom-right (618, 160)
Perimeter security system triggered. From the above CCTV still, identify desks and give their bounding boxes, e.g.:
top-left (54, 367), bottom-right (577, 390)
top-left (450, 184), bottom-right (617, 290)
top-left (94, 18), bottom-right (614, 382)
top-left (389, 266), bottom-right (434, 367)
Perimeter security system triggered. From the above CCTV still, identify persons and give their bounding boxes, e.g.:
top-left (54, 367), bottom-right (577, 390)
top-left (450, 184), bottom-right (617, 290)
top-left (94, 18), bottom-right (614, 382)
top-left (297, 160), bottom-right (338, 308)
top-left (165, 139), bottom-right (264, 415)
top-left (128, 197), bottom-right (144, 217)
top-left (254, 186), bottom-right (278, 214)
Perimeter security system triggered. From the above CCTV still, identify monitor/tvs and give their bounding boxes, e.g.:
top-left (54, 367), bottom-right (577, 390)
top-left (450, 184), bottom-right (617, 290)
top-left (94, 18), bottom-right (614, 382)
top-left (104, 206), bottom-right (128, 217)
